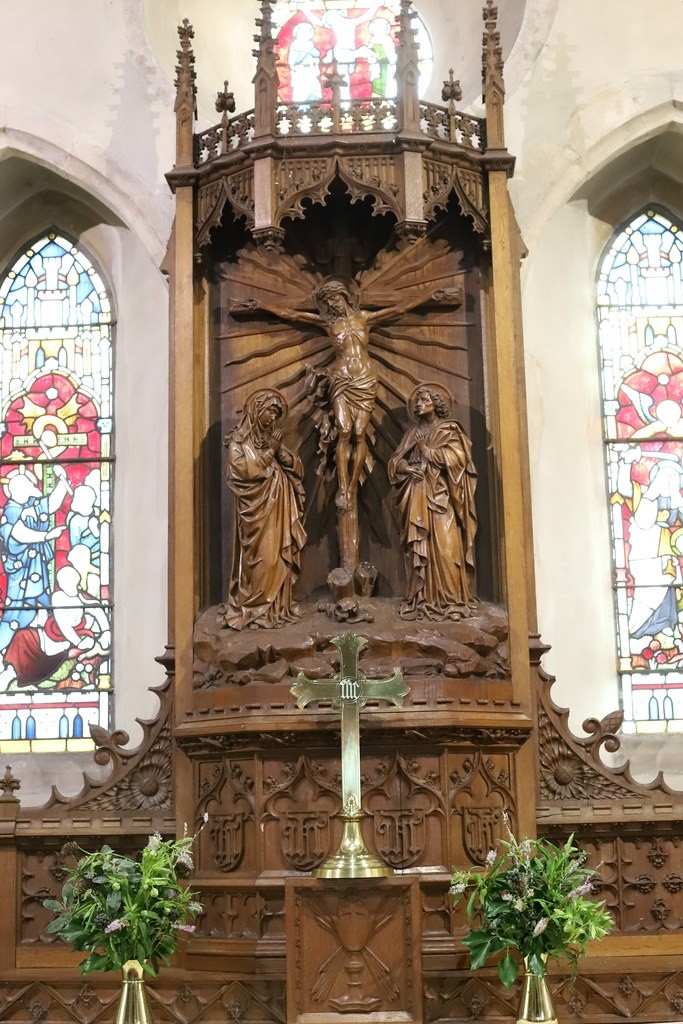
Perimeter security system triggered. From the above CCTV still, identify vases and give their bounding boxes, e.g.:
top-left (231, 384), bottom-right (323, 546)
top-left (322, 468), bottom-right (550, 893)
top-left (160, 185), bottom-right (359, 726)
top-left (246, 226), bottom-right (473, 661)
top-left (515, 952), bottom-right (559, 1024)
top-left (116, 958), bottom-right (153, 1024)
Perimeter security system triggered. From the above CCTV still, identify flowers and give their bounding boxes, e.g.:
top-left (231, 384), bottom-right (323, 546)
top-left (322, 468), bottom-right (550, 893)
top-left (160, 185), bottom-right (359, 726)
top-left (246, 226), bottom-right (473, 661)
top-left (42, 812), bottom-right (209, 981)
top-left (448, 810), bottom-right (617, 991)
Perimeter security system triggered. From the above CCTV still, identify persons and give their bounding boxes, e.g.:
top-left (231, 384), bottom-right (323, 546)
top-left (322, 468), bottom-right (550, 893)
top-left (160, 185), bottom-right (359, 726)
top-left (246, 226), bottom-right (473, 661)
top-left (388, 386), bottom-right (481, 618)
top-left (230, 282), bottom-right (459, 509)
top-left (226, 389), bottom-right (308, 628)
